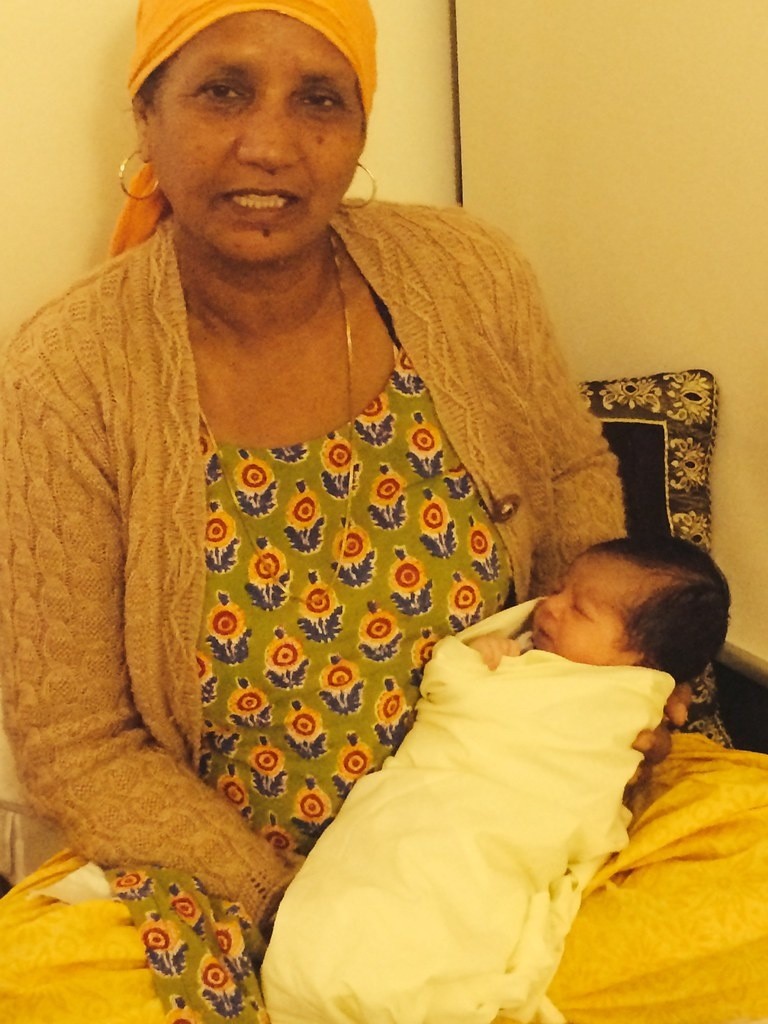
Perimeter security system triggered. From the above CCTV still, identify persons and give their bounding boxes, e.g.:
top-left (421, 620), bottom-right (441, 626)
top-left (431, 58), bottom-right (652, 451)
top-left (0, 0), bottom-right (768, 1024)
top-left (259, 533), bottom-right (734, 1023)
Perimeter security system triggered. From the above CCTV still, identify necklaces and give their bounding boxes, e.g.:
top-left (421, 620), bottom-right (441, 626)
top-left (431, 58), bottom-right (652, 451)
top-left (196, 229), bottom-right (359, 600)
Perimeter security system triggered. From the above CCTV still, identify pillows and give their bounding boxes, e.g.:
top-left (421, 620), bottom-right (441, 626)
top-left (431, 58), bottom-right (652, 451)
top-left (582, 366), bottom-right (733, 751)
